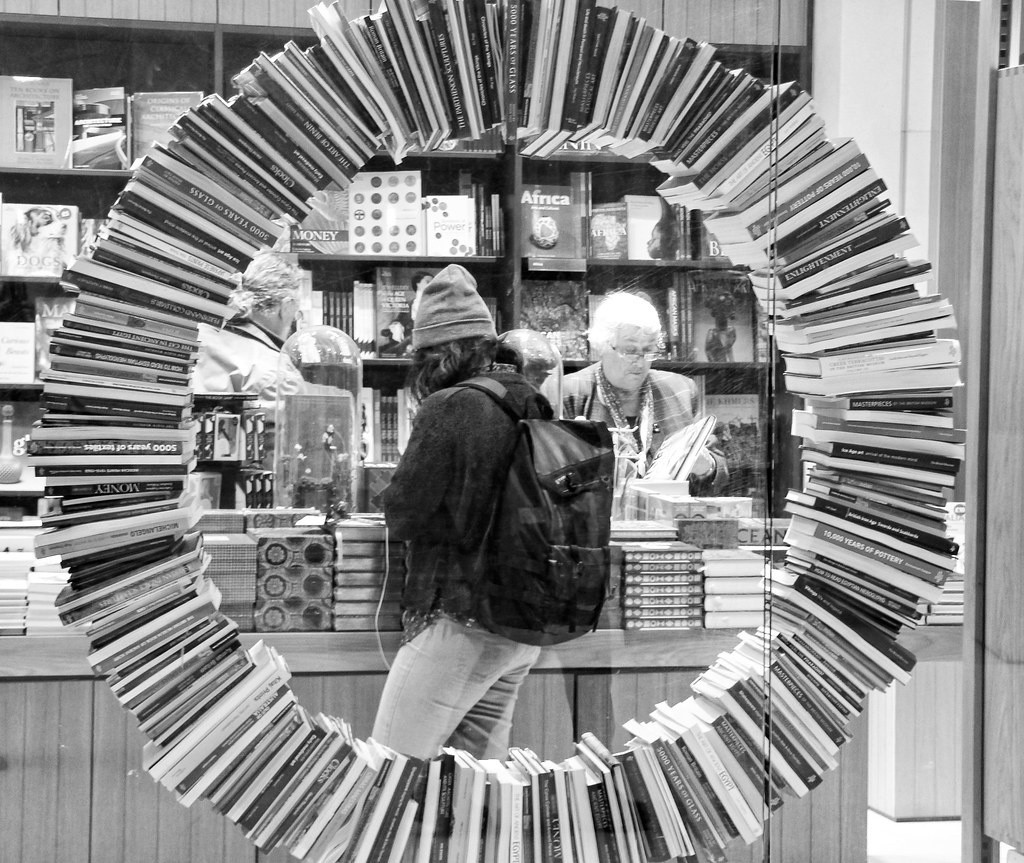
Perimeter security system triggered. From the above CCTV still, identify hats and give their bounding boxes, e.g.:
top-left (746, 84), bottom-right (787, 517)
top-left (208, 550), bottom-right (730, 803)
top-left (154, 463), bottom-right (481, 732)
top-left (412, 264), bottom-right (498, 351)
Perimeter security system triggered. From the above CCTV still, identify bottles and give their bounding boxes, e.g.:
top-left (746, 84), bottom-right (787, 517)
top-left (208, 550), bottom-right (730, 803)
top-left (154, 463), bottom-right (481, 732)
top-left (0, 405), bottom-right (23, 484)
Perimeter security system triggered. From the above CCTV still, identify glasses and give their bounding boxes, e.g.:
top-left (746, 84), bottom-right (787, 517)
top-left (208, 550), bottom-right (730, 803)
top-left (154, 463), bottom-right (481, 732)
top-left (290, 298), bottom-right (302, 322)
top-left (607, 341), bottom-right (660, 363)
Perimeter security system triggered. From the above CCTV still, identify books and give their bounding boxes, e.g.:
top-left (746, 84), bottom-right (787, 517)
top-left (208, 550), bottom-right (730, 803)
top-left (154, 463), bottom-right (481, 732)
top-left (0, 0), bottom-right (967, 863)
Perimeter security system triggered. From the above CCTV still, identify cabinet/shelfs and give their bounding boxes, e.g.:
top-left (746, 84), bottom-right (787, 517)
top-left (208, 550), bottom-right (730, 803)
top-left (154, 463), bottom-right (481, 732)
top-left (0, 13), bottom-right (815, 521)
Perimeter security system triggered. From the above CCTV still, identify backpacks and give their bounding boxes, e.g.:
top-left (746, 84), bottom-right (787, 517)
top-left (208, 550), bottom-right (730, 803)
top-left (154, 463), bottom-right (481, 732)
top-left (459, 376), bottom-right (614, 646)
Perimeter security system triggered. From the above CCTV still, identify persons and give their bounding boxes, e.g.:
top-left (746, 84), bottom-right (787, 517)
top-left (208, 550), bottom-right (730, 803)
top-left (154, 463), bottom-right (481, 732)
top-left (372, 263), bottom-right (552, 759)
top-left (543, 292), bottom-right (729, 497)
top-left (190, 249), bottom-right (305, 471)
